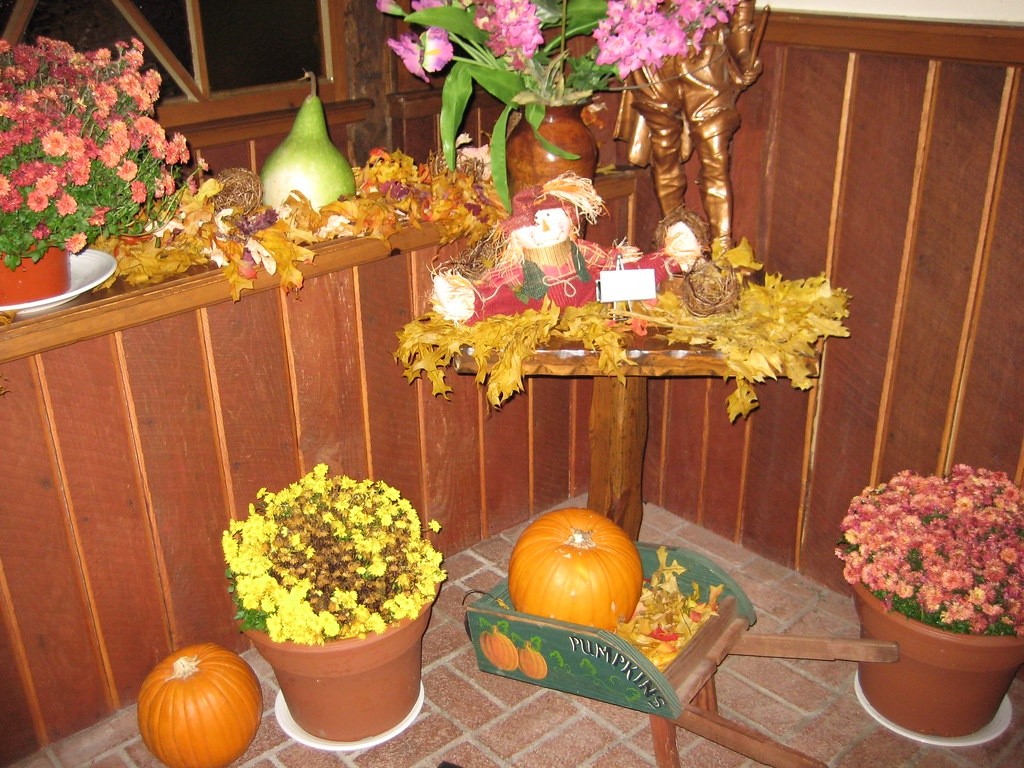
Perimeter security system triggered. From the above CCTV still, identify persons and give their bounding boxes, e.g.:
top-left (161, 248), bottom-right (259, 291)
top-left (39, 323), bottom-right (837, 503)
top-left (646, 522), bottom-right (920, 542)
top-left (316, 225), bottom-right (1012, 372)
top-left (430, 169), bottom-right (704, 320)
top-left (614, 0), bottom-right (762, 276)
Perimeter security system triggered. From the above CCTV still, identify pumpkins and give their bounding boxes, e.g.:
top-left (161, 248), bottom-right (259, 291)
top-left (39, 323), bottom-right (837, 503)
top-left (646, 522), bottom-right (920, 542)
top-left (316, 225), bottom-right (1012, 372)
top-left (138, 642), bottom-right (264, 768)
top-left (508, 507), bottom-right (643, 634)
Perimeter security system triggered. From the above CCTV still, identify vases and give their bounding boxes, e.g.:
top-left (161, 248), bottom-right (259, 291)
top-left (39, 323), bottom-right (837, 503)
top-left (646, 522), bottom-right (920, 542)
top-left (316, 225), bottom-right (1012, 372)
top-left (851, 582), bottom-right (1024, 736)
top-left (0, 246), bottom-right (72, 306)
top-left (242, 585), bottom-right (441, 742)
top-left (505, 101), bottom-right (601, 242)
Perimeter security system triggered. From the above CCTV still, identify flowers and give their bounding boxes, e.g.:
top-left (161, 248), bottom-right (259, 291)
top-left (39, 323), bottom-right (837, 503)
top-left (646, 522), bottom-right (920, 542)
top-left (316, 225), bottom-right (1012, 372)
top-left (376, 0), bottom-right (743, 214)
top-left (221, 462), bottom-right (448, 648)
top-left (0, 37), bottom-right (209, 271)
top-left (833, 463), bottom-right (1023, 639)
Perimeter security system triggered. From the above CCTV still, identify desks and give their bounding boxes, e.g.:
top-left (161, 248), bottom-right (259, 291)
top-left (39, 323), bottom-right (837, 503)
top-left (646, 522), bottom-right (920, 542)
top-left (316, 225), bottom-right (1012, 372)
top-left (449, 329), bottom-right (820, 541)
